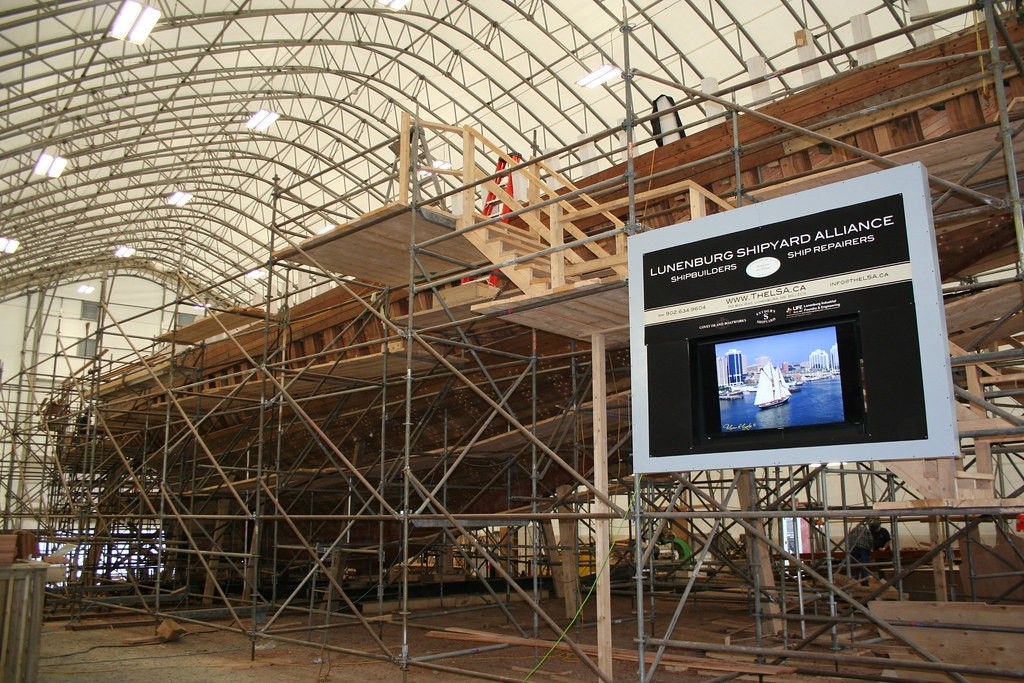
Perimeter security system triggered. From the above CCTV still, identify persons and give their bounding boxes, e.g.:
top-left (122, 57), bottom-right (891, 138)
top-left (78, 412), bottom-right (92, 444)
top-left (848, 523), bottom-right (873, 587)
top-left (872, 525), bottom-right (892, 584)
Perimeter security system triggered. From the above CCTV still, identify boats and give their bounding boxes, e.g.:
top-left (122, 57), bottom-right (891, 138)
top-left (719, 363), bottom-right (835, 408)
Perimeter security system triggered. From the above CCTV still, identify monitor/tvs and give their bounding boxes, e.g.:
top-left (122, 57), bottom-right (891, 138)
top-left (691, 319), bottom-right (865, 440)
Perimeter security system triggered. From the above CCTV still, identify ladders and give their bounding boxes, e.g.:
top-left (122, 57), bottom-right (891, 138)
top-left (460, 152), bottom-right (522, 288)
